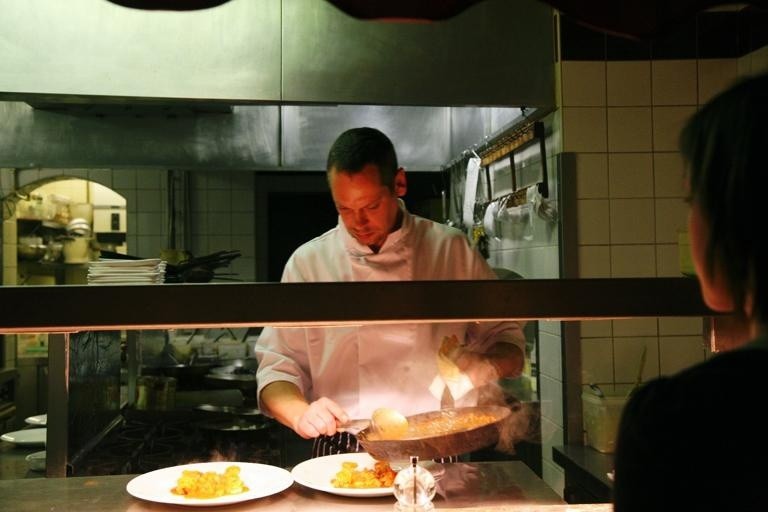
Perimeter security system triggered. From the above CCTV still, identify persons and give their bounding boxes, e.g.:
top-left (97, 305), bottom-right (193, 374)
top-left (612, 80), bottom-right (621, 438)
top-left (254, 129), bottom-right (527, 463)
top-left (613, 72), bottom-right (768, 512)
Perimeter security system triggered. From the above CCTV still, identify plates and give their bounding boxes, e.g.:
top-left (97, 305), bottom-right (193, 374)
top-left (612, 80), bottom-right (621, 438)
top-left (0, 427), bottom-right (47, 449)
top-left (126, 461), bottom-right (294, 507)
top-left (291, 452), bottom-right (445, 499)
top-left (26, 451), bottom-right (46, 471)
top-left (25, 414), bottom-right (48, 426)
top-left (86, 257), bottom-right (169, 285)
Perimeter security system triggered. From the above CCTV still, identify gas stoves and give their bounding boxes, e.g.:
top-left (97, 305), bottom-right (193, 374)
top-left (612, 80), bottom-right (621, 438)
top-left (71, 408), bottom-right (289, 475)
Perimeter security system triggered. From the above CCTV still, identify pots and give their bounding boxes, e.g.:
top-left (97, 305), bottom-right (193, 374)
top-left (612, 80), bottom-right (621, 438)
top-left (203, 356), bottom-right (258, 392)
top-left (194, 401), bottom-right (264, 429)
top-left (141, 364), bottom-right (217, 377)
top-left (335, 406), bottom-right (514, 462)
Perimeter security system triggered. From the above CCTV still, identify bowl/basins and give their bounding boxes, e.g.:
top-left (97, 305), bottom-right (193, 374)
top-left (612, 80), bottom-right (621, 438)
top-left (18, 245), bottom-right (49, 261)
